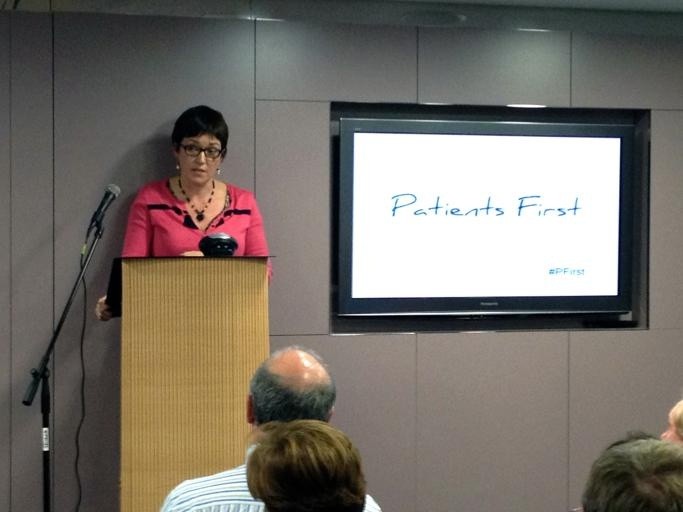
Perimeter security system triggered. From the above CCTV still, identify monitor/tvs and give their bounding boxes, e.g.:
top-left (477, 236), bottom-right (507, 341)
top-left (337, 119), bottom-right (636, 316)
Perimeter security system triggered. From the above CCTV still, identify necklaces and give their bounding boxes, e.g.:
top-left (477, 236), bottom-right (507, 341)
top-left (178, 175), bottom-right (215, 221)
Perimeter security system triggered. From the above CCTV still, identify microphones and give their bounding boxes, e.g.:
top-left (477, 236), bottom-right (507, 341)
top-left (89, 183), bottom-right (121, 231)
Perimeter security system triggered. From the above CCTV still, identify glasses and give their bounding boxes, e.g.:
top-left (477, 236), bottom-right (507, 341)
top-left (177, 143), bottom-right (225, 159)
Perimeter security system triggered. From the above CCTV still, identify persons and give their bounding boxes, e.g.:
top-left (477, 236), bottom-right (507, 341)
top-left (246, 420), bottom-right (367, 512)
top-left (580, 429), bottom-right (683, 512)
top-left (159, 346), bottom-right (383, 512)
top-left (95, 106), bottom-right (273, 322)
top-left (660, 400), bottom-right (683, 441)
